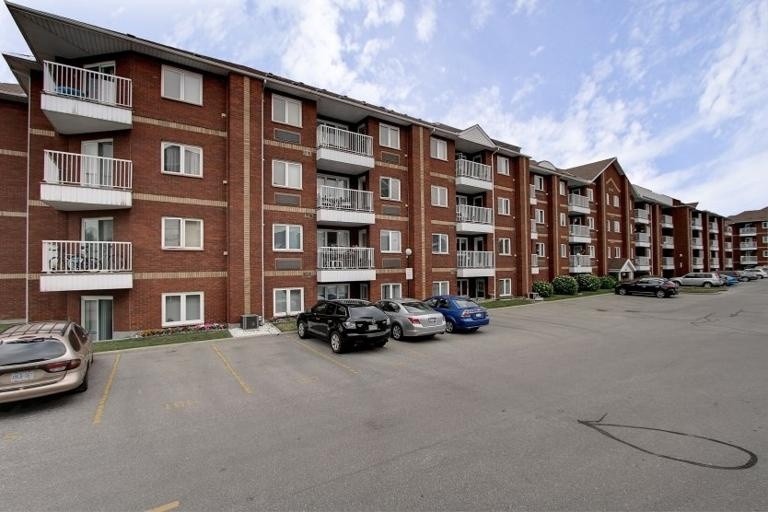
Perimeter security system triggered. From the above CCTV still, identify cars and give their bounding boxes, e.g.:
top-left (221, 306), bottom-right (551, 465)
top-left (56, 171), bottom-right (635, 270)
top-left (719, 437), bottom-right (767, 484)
top-left (718, 265), bottom-right (768, 287)
top-left (296, 297), bottom-right (393, 355)
top-left (422, 294), bottom-right (490, 333)
top-left (373, 298), bottom-right (448, 341)
top-left (1, 319), bottom-right (94, 406)
top-left (669, 272), bottom-right (724, 289)
top-left (614, 275), bottom-right (680, 298)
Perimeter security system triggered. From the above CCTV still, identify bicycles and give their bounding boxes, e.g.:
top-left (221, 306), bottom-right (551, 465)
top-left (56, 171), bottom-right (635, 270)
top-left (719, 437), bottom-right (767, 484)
top-left (47, 243), bottom-right (102, 274)
